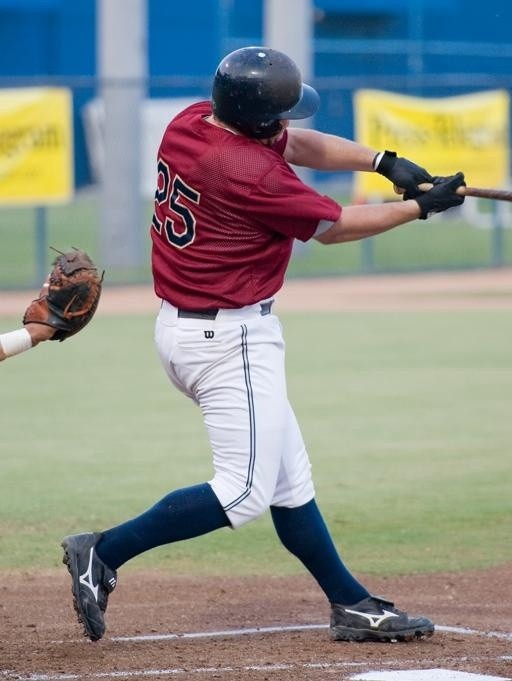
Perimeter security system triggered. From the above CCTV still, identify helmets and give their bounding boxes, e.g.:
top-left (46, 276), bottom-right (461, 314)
top-left (210, 43), bottom-right (322, 140)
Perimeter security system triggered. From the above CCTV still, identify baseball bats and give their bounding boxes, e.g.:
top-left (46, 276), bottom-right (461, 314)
top-left (392, 183), bottom-right (512, 201)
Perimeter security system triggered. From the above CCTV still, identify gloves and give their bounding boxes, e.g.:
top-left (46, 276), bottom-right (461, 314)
top-left (375, 149), bottom-right (435, 200)
top-left (415, 171), bottom-right (467, 220)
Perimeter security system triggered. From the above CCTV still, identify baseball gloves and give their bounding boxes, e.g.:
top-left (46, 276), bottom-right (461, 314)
top-left (22, 246), bottom-right (105, 342)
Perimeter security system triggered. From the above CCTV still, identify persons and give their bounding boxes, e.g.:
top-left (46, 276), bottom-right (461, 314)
top-left (57, 43), bottom-right (467, 646)
top-left (1, 244), bottom-right (107, 362)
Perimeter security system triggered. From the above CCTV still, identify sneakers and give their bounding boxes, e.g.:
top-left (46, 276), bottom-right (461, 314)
top-left (60, 529), bottom-right (118, 643)
top-left (327, 596), bottom-right (433, 641)
